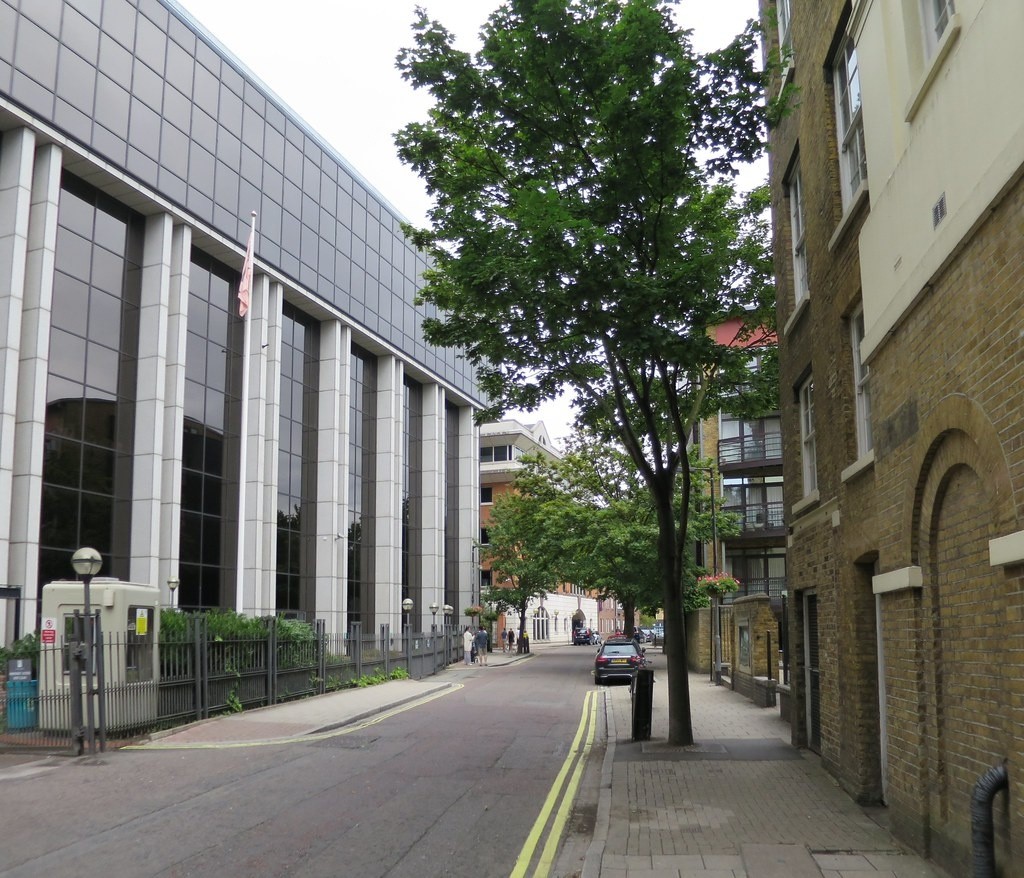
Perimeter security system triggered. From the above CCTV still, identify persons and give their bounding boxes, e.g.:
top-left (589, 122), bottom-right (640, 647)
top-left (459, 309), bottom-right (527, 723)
top-left (464, 627), bottom-right (475, 666)
top-left (501, 628), bottom-right (507, 652)
top-left (506, 628), bottom-right (514, 650)
top-left (476, 626), bottom-right (489, 666)
top-left (616, 626), bottom-right (620, 632)
top-left (516, 627), bottom-right (520, 652)
top-left (523, 630), bottom-right (528, 638)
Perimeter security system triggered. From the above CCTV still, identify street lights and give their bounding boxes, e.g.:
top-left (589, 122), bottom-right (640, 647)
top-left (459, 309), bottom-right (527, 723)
top-left (429, 603), bottom-right (440, 625)
top-left (443, 605), bottom-right (454, 624)
top-left (166, 576), bottom-right (180, 607)
top-left (72, 547), bottom-right (102, 755)
top-left (403, 598), bottom-right (415, 625)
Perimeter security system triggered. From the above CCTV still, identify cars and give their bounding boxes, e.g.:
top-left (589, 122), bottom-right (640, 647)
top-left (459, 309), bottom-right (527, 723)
top-left (595, 638), bottom-right (647, 685)
top-left (633, 627), bottom-right (666, 644)
top-left (572, 627), bottom-right (603, 646)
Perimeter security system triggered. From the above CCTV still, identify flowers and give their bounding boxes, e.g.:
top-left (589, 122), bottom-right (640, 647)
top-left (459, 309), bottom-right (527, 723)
top-left (693, 572), bottom-right (742, 596)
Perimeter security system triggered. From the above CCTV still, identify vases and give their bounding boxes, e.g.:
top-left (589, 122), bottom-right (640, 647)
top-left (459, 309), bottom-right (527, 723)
top-left (705, 589), bottom-right (728, 599)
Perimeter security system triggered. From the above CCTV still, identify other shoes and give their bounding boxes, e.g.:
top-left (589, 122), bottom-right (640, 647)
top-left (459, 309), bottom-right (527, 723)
top-left (485, 664), bottom-right (487, 666)
top-left (468, 664), bottom-right (472, 665)
top-left (480, 665), bottom-right (482, 666)
